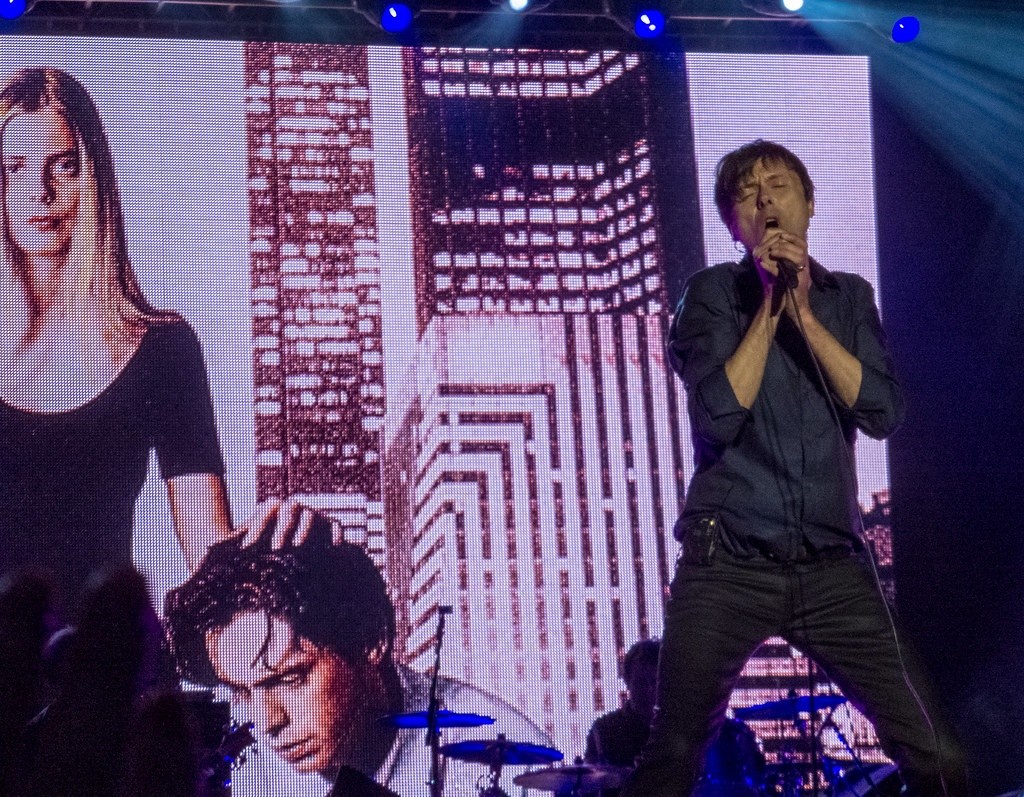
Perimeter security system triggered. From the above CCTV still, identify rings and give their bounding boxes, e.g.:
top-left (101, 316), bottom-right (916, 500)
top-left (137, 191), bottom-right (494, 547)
top-left (796, 266), bottom-right (805, 270)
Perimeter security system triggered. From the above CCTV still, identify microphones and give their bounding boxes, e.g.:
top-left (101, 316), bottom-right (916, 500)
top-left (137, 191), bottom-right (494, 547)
top-left (776, 257), bottom-right (799, 289)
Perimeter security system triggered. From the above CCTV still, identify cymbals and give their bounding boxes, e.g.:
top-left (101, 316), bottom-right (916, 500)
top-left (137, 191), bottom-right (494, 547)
top-left (378, 710), bottom-right (635, 797)
top-left (732, 695), bottom-right (848, 721)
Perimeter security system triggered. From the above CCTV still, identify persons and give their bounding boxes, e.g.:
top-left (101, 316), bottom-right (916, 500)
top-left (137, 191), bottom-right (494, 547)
top-left (0, 66), bottom-right (345, 797)
top-left (0, 564), bottom-right (195, 797)
top-left (618, 138), bottom-right (975, 797)
top-left (583, 637), bottom-right (772, 797)
top-left (164, 531), bottom-right (564, 797)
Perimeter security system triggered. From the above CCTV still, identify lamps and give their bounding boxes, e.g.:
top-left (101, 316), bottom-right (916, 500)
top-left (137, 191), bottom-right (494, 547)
top-left (603, 0), bottom-right (670, 41)
top-left (775, 0), bottom-right (806, 12)
top-left (861, 5), bottom-right (922, 46)
top-left (353, 0), bottom-right (414, 35)
